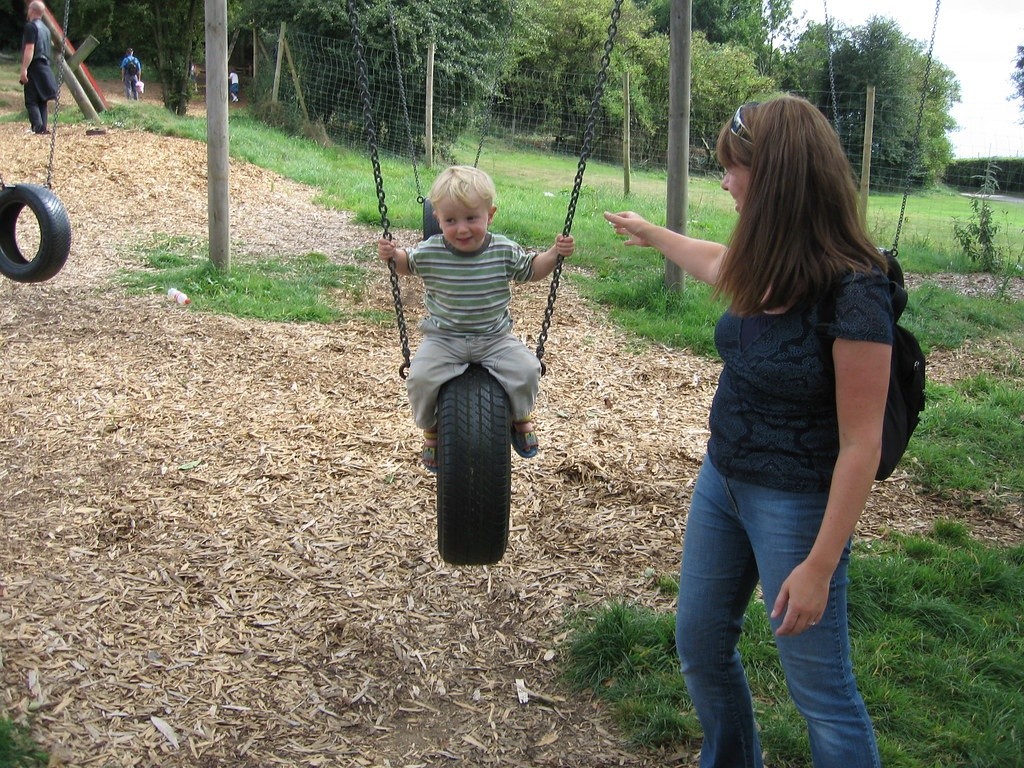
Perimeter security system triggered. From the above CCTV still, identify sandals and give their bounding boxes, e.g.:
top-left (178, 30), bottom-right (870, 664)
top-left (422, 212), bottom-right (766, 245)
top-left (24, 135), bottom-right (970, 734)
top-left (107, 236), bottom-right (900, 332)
top-left (421, 430), bottom-right (438, 471)
top-left (510, 414), bottom-right (538, 458)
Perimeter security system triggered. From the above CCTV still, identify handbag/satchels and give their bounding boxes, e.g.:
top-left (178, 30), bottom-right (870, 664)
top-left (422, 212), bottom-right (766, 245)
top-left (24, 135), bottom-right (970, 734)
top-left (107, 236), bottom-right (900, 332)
top-left (136, 81), bottom-right (144, 93)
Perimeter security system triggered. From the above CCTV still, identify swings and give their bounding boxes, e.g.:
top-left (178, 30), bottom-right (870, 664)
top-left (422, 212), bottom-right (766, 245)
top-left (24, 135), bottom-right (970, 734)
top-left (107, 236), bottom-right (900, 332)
top-left (344, 1), bottom-right (628, 567)
top-left (388, 2), bottom-right (516, 242)
top-left (0, 2), bottom-right (72, 283)
top-left (823, 0), bottom-right (942, 287)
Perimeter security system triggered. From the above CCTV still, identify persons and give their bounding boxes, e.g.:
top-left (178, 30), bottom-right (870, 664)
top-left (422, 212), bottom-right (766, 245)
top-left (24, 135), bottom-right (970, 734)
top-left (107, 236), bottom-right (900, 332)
top-left (227, 71), bottom-right (239, 103)
top-left (190, 63), bottom-right (198, 92)
top-left (378, 165), bottom-right (575, 469)
top-left (601, 93), bottom-right (898, 765)
top-left (19, 1), bottom-right (55, 134)
top-left (121, 48), bottom-right (141, 102)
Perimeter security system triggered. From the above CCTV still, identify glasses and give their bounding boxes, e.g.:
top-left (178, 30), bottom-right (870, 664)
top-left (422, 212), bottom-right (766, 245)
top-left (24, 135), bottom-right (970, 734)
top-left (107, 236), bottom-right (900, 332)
top-left (731, 102), bottom-right (757, 145)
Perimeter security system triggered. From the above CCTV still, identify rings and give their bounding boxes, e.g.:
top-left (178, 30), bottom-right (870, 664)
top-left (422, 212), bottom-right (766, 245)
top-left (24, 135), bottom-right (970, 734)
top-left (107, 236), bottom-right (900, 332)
top-left (807, 622), bottom-right (816, 625)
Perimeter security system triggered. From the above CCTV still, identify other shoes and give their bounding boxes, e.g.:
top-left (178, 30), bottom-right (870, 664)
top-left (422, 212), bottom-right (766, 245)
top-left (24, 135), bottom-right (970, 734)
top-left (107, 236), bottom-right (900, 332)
top-left (232, 96), bottom-right (238, 102)
top-left (32, 125), bottom-right (51, 134)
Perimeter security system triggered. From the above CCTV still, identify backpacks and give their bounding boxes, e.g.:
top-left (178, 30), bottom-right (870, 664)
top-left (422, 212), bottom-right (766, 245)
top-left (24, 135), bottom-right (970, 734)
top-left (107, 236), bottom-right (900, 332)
top-left (126, 57), bottom-right (138, 76)
top-left (819, 250), bottom-right (926, 481)
top-left (193, 64), bottom-right (199, 78)
